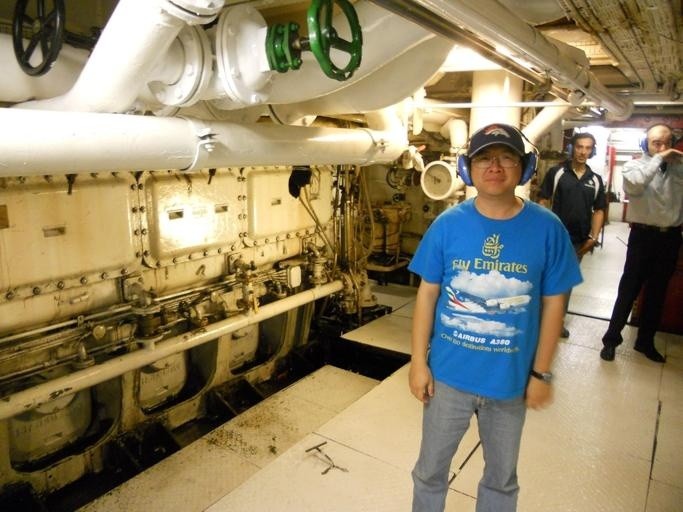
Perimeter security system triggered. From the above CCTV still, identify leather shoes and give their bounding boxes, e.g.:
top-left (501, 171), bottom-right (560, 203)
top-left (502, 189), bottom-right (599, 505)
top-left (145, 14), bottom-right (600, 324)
top-left (634, 345), bottom-right (665, 362)
top-left (601, 345), bottom-right (614, 360)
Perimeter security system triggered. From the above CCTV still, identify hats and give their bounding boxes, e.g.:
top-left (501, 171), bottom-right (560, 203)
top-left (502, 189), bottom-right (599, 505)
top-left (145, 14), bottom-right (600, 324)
top-left (468, 123), bottom-right (532, 160)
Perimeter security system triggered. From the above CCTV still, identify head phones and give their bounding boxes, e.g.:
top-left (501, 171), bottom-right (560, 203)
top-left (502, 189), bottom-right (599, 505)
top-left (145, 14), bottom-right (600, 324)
top-left (456, 123), bottom-right (540, 187)
top-left (566, 133), bottom-right (596, 158)
top-left (639, 124), bottom-right (675, 152)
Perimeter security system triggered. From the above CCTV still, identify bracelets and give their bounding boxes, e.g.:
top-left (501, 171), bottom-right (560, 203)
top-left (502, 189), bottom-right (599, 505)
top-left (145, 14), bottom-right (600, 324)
top-left (530, 370), bottom-right (553, 383)
top-left (588, 234), bottom-right (598, 245)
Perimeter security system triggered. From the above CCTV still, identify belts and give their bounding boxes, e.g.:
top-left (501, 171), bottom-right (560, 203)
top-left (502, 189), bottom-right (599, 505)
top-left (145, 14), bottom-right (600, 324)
top-left (631, 223), bottom-right (681, 232)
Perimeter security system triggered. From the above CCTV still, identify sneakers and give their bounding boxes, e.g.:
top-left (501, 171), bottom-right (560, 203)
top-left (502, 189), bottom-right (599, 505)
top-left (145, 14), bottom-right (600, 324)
top-left (561, 327), bottom-right (568, 337)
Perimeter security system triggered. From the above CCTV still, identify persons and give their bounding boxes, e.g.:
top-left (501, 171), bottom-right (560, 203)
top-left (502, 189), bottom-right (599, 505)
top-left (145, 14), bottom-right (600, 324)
top-left (536, 132), bottom-right (606, 338)
top-left (405, 122), bottom-right (583, 512)
top-left (599, 123), bottom-right (682, 363)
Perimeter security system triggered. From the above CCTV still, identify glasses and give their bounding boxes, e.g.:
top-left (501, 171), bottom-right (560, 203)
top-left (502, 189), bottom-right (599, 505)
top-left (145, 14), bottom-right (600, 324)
top-left (472, 155), bottom-right (521, 168)
